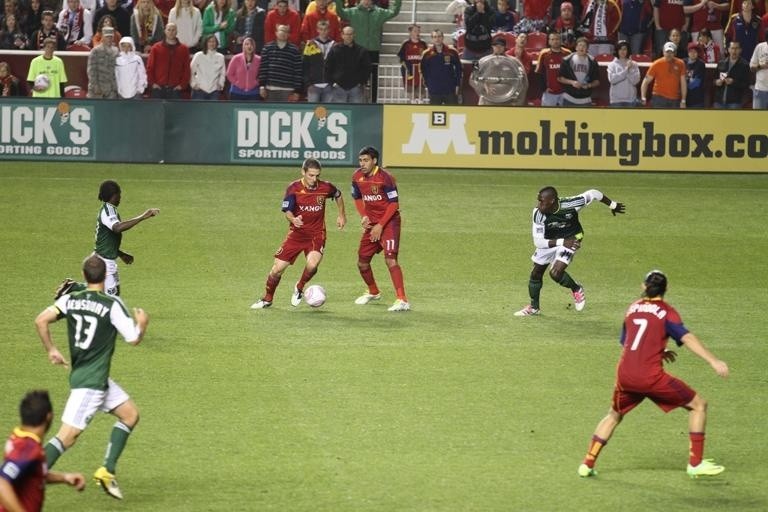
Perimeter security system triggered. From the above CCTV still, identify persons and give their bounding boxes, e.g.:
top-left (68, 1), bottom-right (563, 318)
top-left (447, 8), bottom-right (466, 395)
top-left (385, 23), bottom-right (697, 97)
top-left (2, 389), bottom-right (86, 512)
top-left (352, 146), bottom-right (410, 310)
top-left (579, 267), bottom-right (737, 481)
top-left (512, 184), bottom-right (624, 318)
top-left (246, 157), bottom-right (346, 310)
top-left (87, 177), bottom-right (163, 299)
top-left (30, 252), bottom-right (151, 501)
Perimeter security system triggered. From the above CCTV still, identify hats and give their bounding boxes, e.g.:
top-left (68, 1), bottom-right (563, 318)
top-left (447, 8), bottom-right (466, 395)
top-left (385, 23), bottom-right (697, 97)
top-left (491, 36), bottom-right (508, 46)
top-left (687, 42), bottom-right (698, 51)
top-left (663, 42), bottom-right (677, 53)
top-left (560, 1), bottom-right (572, 11)
top-left (616, 39), bottom-right (629, 48)
top-left (101, 26), bottom-right (115, 37)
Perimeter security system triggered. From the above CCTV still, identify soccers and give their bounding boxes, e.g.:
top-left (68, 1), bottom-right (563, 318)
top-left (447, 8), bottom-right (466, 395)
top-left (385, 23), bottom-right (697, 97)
top-left (35, 73), bottom-right (50, 90)
top-left (304, 285), bottom-right (327, 306)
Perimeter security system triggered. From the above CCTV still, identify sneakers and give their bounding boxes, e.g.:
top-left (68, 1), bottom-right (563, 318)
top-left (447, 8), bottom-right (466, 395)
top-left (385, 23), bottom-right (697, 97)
top-left (250, 298), bottom-right (273, 309)
top-left (578, 463), bottom-right (598, 477)
top-left (571, 284), bottom-right (586, 312)
top-left (387, 299), bottom-right (409, 311)
top-left (53, 278), bottom-right (75, 301)
top-left (513, 304), bottom-right (541, 316)
top-left (686, 457), bottom-right (725, 479)
top-left (92, 466), bottom-right (124, 500)
top-left (354, 289), bottom-right (381, 305)
top-left (290, 280), bottom-right (304, 307)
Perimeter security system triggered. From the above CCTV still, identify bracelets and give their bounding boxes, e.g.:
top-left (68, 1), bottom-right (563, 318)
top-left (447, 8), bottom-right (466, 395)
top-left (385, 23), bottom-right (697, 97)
top-left (608, 201), bottom-right (618, 211)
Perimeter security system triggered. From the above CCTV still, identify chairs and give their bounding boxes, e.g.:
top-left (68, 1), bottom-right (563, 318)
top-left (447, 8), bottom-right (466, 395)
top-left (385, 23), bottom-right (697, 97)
top-left (63, 43), bottom-right (306, 103)
top-left (465, 0), bottom-right (767, 109)
